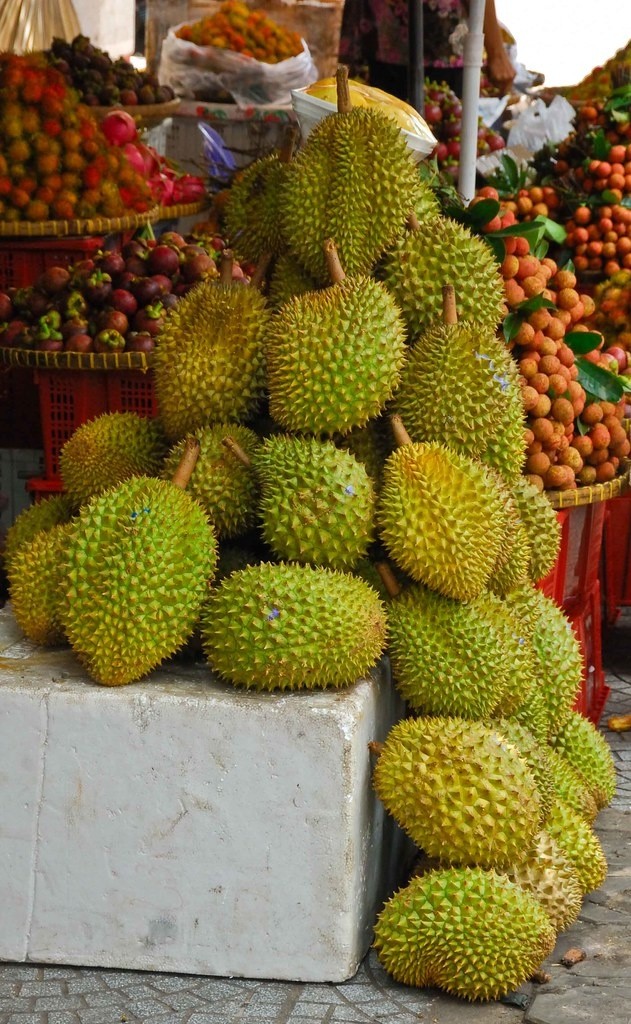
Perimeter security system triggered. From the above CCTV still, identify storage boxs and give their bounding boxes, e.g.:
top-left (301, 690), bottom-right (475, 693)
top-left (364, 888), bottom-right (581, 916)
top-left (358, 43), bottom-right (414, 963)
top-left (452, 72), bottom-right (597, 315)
top-left (1, 597), bottom-right (420, 986)
top-left (0, 235), bottom-right (107, 293)
top-left (27, 367), bottom-right (158, 504)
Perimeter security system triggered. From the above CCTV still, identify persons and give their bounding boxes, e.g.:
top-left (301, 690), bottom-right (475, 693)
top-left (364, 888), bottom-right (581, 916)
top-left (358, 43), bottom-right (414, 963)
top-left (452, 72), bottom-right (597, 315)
top-left (338, 0), bottom-right (513, 101)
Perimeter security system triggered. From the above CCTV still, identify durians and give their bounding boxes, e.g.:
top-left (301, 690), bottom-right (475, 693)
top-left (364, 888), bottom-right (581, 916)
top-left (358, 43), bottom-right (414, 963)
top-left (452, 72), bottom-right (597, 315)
top-left (155, 60), bottom-right (617, 1003)
top-left (0, 410), bottom-right (219, 687)
